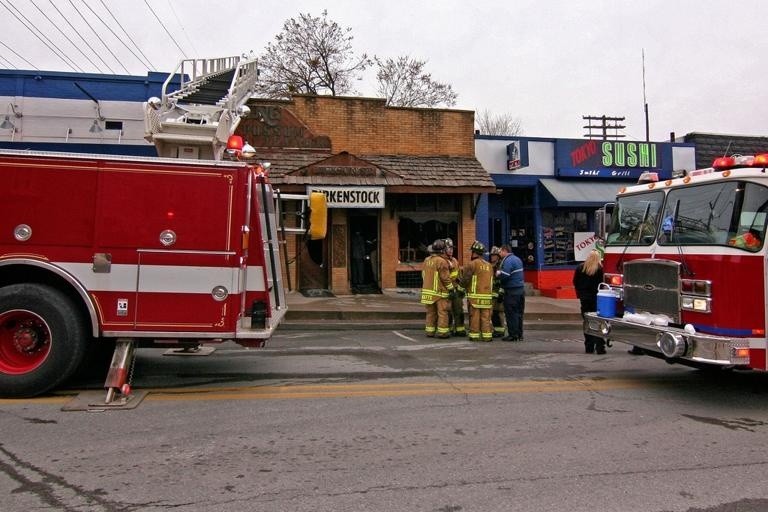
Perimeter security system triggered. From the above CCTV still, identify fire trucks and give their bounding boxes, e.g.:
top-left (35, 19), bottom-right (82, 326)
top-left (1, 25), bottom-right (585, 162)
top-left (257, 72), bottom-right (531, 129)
top-left (0, 135), bottom-right (288, 399)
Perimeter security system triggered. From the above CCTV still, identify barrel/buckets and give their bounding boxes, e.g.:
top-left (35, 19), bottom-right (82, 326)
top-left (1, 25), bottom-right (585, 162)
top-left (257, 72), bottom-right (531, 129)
top-left (596, 282), bottom-right (616, 317)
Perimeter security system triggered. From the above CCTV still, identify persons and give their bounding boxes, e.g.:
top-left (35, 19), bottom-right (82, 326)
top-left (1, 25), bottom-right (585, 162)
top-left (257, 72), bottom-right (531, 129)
top-left (494, 244), bottom-right (525, 341)
top-left (573, 250), bottom-right (607, 354)
top-left (421, 239), bottom-right (457, 338)
top-left (455, 240), bottom-right (495, 343)
top-left (489, 246), bottom-right (506, 338)
top-left (440, 237), bottom-right (466, 336)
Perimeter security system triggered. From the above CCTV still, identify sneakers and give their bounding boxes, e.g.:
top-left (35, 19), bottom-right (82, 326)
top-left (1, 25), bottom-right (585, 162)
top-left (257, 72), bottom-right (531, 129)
top-left (469, 331), bottom-right (504, 342)
top-left (426, 331), bottom-right (465, 338)
top-left (585, 349), bottom-right (607, 354)
top-left (505, 335), bottom-right (523, 340)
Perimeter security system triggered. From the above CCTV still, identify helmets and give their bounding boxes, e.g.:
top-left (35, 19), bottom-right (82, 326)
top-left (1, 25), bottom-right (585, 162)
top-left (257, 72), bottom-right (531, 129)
top-left (432, 238), bottom-right (454, 251)
top-left (490, 246), bottom-right (500, 254)
top-left (471, 240), bottom-right (486, 252)
